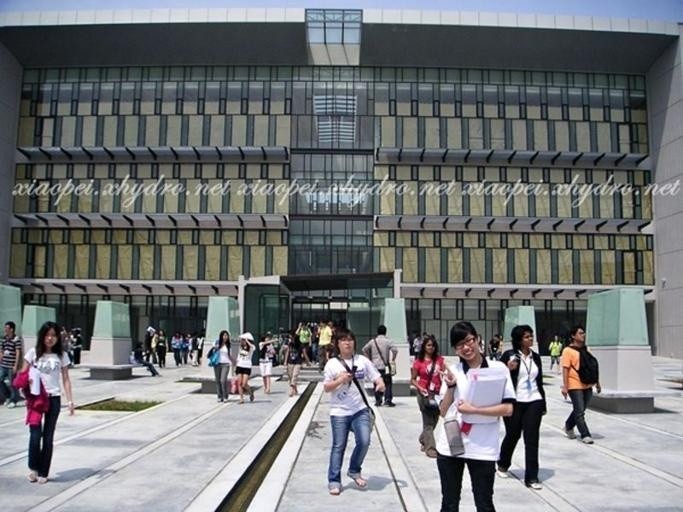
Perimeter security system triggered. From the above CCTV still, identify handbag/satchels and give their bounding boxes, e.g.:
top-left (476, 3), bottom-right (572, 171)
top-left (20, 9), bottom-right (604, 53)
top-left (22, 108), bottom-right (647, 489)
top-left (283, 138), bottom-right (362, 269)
top-left (385, 359), bottom-right (396, 376)
top-left (350, 407), bottom-right (375, 432)
top-left (420, 390), bottom-right (440, 415)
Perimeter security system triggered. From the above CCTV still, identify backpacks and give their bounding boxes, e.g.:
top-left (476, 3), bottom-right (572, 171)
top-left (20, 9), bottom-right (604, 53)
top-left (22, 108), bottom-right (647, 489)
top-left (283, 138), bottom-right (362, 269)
top-left (568, 344), bottom-right (599, 384)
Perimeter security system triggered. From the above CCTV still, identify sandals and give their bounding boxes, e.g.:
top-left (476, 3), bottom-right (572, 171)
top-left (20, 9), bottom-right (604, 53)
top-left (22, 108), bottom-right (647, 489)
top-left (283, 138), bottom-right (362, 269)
top-left (355, 477), bottom-right (367, 487)
top-left (329, 484), bottom-right (341, 495)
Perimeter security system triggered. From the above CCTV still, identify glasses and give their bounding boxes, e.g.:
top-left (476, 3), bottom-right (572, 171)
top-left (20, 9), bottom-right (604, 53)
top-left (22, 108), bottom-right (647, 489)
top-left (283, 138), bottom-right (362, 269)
top-left (456, 336), bottom-right (476, 350)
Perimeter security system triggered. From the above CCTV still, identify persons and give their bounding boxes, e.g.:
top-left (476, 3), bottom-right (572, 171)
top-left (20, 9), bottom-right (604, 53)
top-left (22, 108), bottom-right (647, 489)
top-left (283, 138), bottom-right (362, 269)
top-left (134, 320), bottom-right (338, 404)
top-left (60, 327), bottom-right (82, 364)
top-left (549, 336), bottom-right (563, 373)
top-left (477, 334), bottom-right (504, 361)
top-left (408, 332), bottom-right (439, 360)
top-left (324, 329), bottom-right (386, 494)
top-left (495, 325), bottom-right (547, 490)
top-left (437, 321), bottom-right (516, 512)
top-left (411, 334), bottom-right (447, 458)
top-left (362, 325), bottom-right (398, 407)
top-left (561, 326), bottom-right (602, 445)
top-left (0, 321), bottom-right (23, 408)
top-left (13, 321), bottom-right (75, 485)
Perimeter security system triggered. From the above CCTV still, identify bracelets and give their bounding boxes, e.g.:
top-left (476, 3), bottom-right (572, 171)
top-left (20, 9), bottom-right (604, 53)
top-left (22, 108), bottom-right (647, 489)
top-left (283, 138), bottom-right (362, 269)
top-left (68, 401), bottom-right (74, 406)
top-left (447, 383), bottom-right (457, 388)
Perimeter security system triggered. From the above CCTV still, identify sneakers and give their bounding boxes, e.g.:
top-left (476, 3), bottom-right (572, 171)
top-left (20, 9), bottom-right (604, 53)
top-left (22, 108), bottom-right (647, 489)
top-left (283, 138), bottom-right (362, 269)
top-left (37, 477), bottom-right (47, 483)
top-left (583, 436), bottom-right (594, 443)
top-left (565, 428), bottom-right (577, 439)
top-left (28, 470), bottom-right (37, 481)
top-left (384, 399), bottom-right (395, 406)
top-left (495, 462), bottom-right (508, 478)
top-left (528, 480), bottom-right (542, 489)
top-left (375, 401), bottom-right (382, 406)
top-left (427, 449), bottom-right (438, 457)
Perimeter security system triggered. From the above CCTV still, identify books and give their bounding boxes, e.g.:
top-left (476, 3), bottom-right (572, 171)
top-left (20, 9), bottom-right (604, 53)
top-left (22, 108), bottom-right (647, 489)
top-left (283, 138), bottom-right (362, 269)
top-left (461, 368), bottom-right (507, 424)
